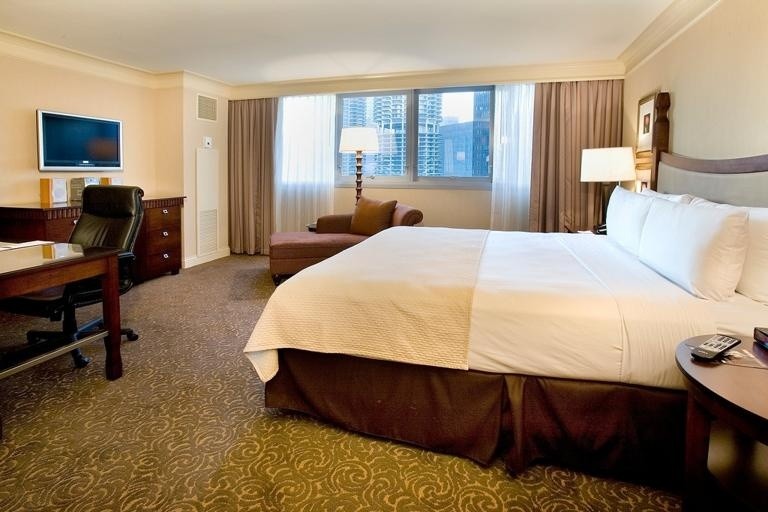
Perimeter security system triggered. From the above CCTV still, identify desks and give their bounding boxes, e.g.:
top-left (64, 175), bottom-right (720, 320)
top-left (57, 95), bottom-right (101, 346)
top-left (0, 241), bottom-right (123, 382)
top-left (0, 196), bottom-right (186, 320)
top-left (676, 335), bottom-right (768, 512)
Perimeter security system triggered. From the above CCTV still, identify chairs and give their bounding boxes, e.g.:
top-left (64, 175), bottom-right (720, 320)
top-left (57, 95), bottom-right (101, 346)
top-left (1, 185), bottom-right (145, 367)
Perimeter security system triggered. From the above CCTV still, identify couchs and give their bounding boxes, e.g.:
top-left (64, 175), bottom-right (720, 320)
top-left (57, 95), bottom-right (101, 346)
top-left (270, 204), bottom-right (422, 287)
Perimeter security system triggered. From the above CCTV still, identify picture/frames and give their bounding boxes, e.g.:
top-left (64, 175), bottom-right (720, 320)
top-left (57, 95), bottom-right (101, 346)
top-left (635, 92), bottom-right (659, 156)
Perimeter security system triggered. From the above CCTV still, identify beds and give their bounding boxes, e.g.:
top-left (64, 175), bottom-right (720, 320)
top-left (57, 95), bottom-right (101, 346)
top-left (243, 92), bottom-right (768, 480)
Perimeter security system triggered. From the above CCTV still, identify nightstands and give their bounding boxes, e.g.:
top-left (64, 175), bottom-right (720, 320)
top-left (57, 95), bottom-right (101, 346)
top-left (565, 224), bottom-right (606, 234)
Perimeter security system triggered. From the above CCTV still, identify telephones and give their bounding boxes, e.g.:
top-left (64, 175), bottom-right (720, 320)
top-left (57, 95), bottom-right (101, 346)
top-left (598, 224), bottom-right (606, 234)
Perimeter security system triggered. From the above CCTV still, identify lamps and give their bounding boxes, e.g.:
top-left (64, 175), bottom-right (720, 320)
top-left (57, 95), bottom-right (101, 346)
top-left (338, 128), bottom-right (380, 208)
top-left (581, 147), bottom-right (636, 235)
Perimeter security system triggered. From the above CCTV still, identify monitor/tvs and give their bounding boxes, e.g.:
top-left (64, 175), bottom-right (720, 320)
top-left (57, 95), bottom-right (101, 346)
top-left (36, 109), bottom-right (123, 172)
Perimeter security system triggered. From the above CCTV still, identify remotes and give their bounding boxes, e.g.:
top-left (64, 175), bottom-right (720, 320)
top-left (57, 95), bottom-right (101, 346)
top-left (691, 334), bottom-right (741, 361)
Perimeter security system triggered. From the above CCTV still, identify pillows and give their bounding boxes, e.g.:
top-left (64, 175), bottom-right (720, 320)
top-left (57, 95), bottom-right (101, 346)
top-left (350, 197), bottom-right (397, 234)
top-left (606, 185), bottom-right (655, 257)
top-left (637, 198), bottom-right (748, 302)
top-left (642, 188), bottom-right (692, 205)
top-left (689, 197), bottom-right (768, 306)
